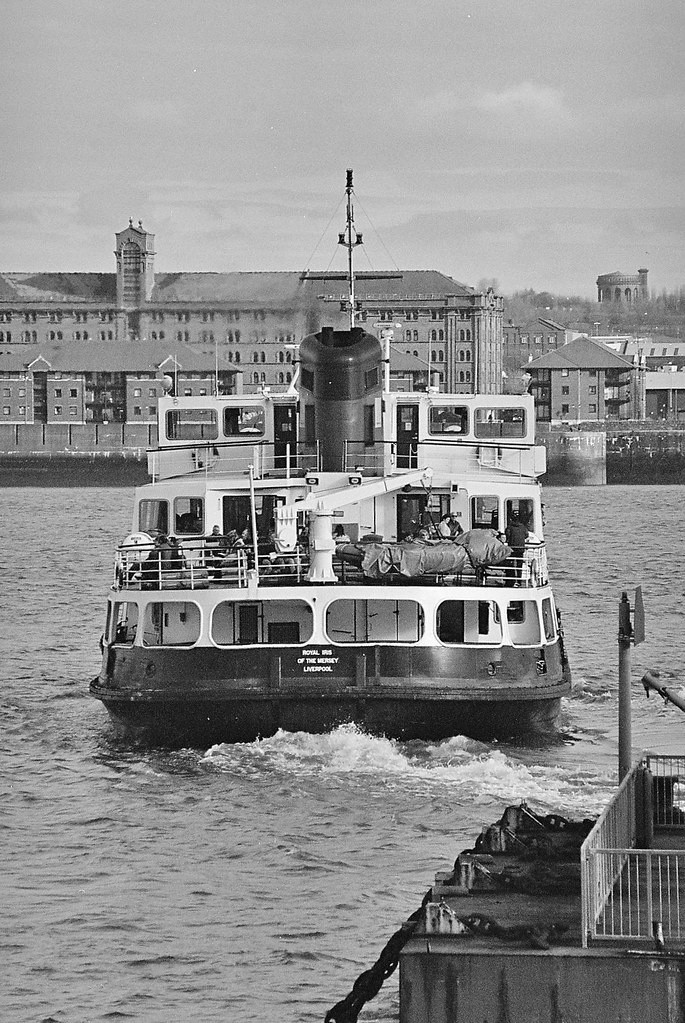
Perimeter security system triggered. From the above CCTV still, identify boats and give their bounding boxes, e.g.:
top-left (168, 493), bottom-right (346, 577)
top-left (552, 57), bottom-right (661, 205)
top-left (85, 169), bottom-right (575, 751)
top-left (323, 584), bottom-right (684, 1023)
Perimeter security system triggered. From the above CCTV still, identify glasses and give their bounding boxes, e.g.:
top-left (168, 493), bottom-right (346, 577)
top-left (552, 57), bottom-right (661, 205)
top-left (451, 515), bottom-right (457, 517)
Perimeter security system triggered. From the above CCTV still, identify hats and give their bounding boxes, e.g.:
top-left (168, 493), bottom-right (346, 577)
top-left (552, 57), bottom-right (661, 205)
top-left (227, 529), bottom-right (237, 538)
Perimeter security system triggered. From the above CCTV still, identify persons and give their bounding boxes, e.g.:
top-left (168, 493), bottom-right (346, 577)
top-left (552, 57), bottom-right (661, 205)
top-left (332, 525), bottom-right (351, 543)
top-left (505, 513), bottom-right (529, 587)
top-left (438, 512), bottom-right (464, 536)
top-left (206, 526), bottom-right (224, 584)
top-left (228, 528), bottom-right (252, 554)
top-left (150, 535), bottom-right (179, 580)
